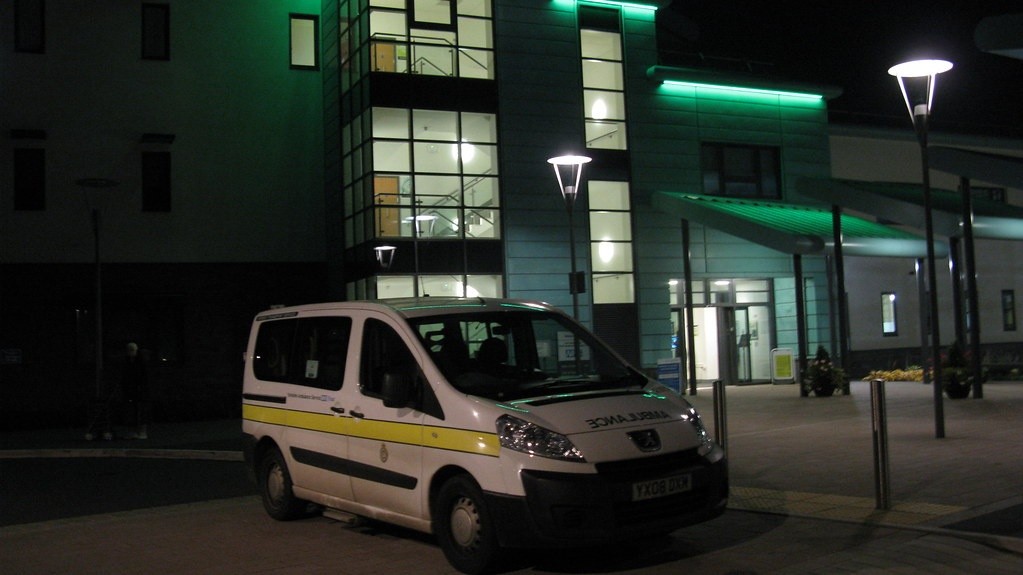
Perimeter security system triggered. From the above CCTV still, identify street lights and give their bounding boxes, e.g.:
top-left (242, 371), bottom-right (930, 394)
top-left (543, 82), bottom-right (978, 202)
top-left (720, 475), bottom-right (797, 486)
top-left (75, 177), bottom-right (119, 397)
top-left (544, 147), bottom-right (595, 376)
top-left (886, 57), bottom-right (955, 441)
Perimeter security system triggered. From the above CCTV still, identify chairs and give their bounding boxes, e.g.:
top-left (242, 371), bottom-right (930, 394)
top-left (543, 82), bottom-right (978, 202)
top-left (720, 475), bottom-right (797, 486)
top-left (475, 338), bottom-right (529, 402)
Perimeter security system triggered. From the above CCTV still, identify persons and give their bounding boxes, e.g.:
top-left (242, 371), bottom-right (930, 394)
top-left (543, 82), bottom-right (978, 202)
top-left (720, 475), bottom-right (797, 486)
top-left (122, 342), bottom-right (149, 440)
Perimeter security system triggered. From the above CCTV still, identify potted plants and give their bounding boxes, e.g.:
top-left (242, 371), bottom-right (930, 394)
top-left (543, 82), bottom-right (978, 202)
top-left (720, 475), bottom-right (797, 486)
top-left (937, 367), bottom-right (977, 401)
top-left (800, 361), bottom-right (846, 399)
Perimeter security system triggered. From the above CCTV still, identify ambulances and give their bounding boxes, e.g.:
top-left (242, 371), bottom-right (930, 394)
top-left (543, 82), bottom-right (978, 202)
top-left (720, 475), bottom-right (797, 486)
top-left (238, 294), bottom-right (731, 575)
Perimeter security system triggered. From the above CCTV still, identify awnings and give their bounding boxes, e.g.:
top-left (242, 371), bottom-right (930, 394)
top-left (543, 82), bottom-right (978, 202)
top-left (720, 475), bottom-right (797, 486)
top-left (653, 190), bottom-right (1023, 260)
